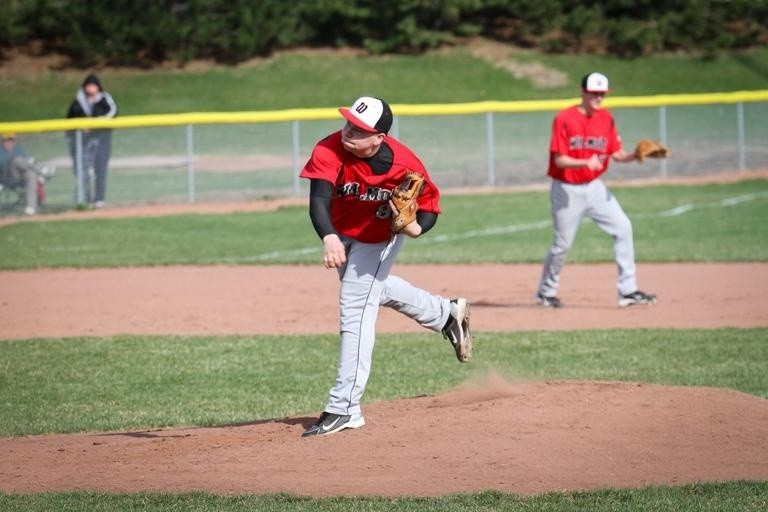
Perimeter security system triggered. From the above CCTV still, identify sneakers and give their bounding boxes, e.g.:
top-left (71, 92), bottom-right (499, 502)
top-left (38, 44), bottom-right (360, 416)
top-left (442, 298), bottom-right (474, 363)
top-left (618, 290), bottom-right (657, 308)
top-left (301, 411), bottom-right (365, 438)
top-left (536, 294), bottom-right (564, 308)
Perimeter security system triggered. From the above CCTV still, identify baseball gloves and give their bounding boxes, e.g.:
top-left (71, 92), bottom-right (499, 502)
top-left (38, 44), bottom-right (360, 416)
top-left (635, 140), bottom-right (669, 166)
top-left (388, 171), bottom-right (425, 234)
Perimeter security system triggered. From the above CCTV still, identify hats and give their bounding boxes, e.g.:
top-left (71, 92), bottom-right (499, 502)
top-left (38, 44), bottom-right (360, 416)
top-left (338, 95), bottom-right (393, 135)
top-left (582, 72), bottom-right (611, 94)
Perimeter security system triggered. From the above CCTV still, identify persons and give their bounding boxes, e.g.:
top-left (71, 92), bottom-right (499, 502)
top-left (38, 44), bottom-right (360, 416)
top-left (533, 69), bottom-right (674, 312)
top-left (63, 72), bottom-right (119, 209)
top-left (298, 92), bottom-right (471, 440)
top-left (0, 131), bottom-right (58, 220)
